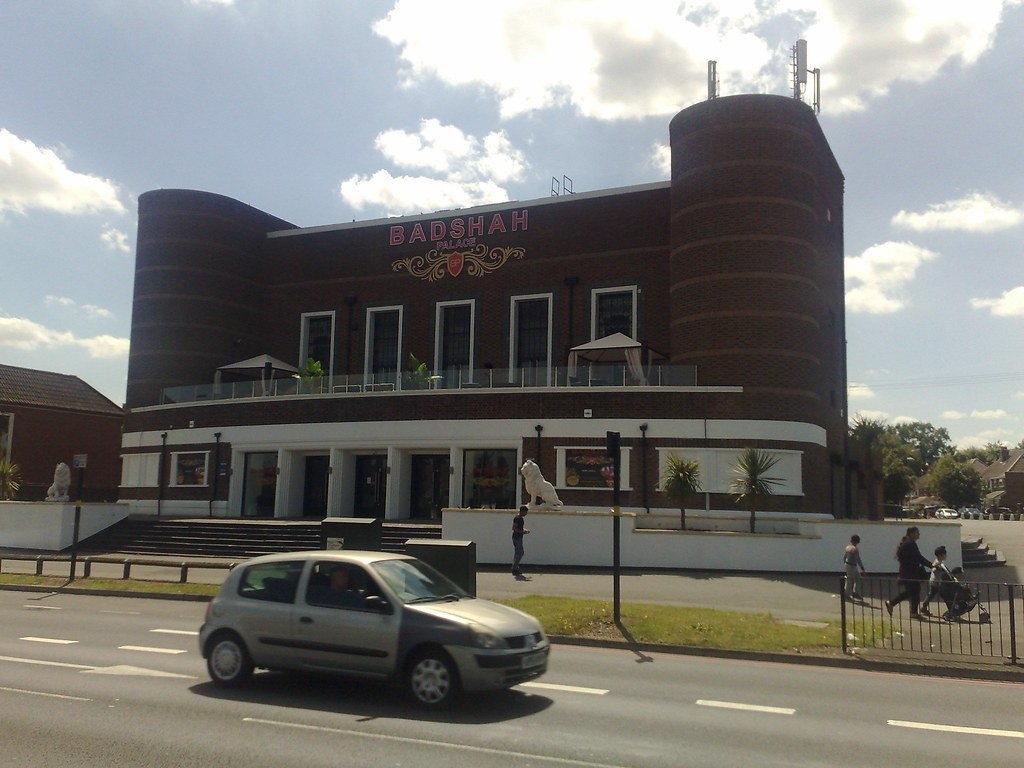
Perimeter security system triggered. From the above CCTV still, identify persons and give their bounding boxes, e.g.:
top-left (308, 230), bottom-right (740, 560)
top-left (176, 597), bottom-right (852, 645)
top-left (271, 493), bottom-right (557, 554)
top-left (319, 566), bottom-right (361, 609)
top-left (885, 527), bottom-right (944, 619)
top-left (953, 567), bottom-right (965, 585)
top-left (510, 506), bottom-right (532, 576)
top-left (921, 546), bottom-right (950, 618)
top-left (843, 534), bottom-right (867, 602)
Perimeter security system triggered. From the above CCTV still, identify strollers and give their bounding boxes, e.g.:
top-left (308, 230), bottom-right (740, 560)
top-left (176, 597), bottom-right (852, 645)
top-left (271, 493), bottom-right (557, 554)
top-left (932, 562), bottom-right (990, 624)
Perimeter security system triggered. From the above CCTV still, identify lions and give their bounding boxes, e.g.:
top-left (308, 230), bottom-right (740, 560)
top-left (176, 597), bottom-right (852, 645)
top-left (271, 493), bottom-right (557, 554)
top-left (48, 462), bottom-right (71, 497)
top-left (521, 459), bottom-right (563, 510)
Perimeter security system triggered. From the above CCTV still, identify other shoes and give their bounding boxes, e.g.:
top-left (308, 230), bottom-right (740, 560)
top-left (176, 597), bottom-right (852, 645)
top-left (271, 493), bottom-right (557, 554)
top-left (845, 597), bottom-right (855, 603)
top-left (921, 608), bottom-right (933, 618)
top-left (511, 568), bottom-right (523, 575)
top-left (910, 613), bottom-right (927, 622)
top-left (885, 601), bottom-right (892, 616)
top-left (950, 616), bottom-right (965, 622)
top-left (853, 593), bottom-right (863, 601)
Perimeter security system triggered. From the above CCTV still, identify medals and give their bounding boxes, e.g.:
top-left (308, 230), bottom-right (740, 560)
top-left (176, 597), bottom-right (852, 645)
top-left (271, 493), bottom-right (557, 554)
top-left (212, 353), bottom-right (306, 398)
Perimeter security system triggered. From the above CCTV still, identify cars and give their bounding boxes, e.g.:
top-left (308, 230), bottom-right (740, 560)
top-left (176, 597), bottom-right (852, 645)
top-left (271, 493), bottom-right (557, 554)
top-left (935, 508), bottom-right (959, 520)
top-left (197, 550), bottom-right (550, 712)
top-left (986, 506), bottom-right (1013, 520)
top-left (961, 507), bottom-right (986, 519)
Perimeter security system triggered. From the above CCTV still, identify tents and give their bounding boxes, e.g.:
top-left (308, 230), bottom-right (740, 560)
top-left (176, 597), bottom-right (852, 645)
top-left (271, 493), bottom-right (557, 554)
top-left (568, 332), bottom-right (669, 385)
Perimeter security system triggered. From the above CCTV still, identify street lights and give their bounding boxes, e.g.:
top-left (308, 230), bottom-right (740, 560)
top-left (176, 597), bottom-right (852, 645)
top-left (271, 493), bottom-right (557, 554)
top-left (637, 424), bottom-right (654, 513)
top-left (535, 423), bottom-right (546, 506)
top-left (205, 431), bottom-right (225, 518)
top-left (156, 431), bottom-right (171, 516)
